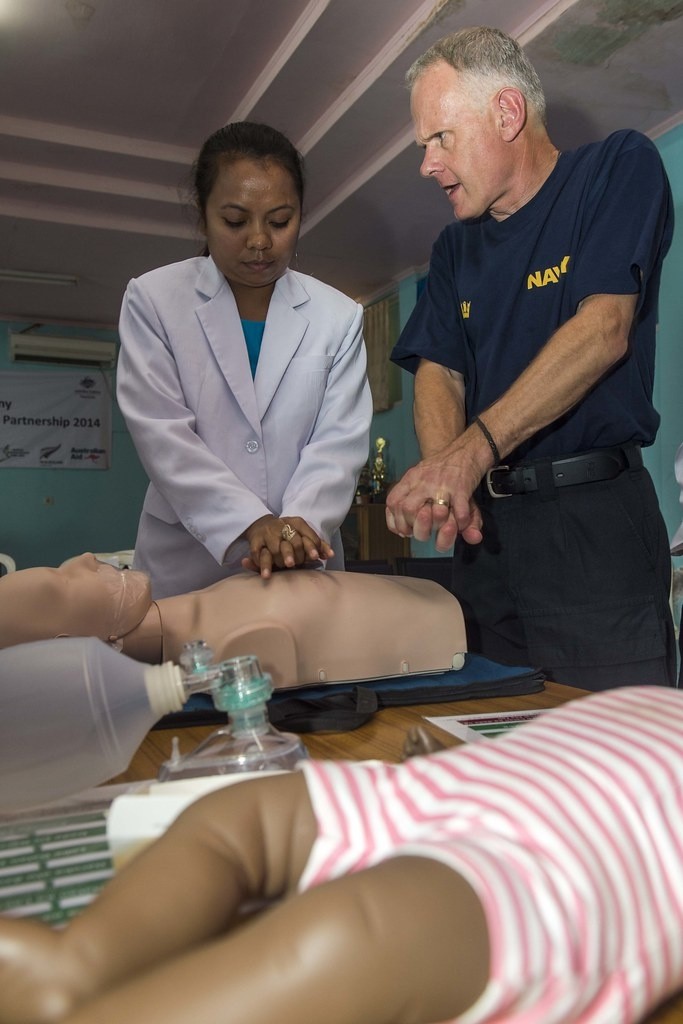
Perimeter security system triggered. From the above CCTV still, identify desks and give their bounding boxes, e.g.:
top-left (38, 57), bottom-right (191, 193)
top-left (0, 679), bottom-right (683, 1024)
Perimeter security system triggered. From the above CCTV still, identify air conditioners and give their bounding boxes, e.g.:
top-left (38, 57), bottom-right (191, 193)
top-left (8, 331), bottom-right (118, 369)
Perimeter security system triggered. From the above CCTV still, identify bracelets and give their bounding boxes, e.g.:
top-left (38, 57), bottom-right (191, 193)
top-left (473, 415), bottom-right (500, 469)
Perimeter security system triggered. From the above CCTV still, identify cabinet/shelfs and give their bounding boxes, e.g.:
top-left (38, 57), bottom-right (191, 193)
top-left (340, 503), bottom-right (411, 575)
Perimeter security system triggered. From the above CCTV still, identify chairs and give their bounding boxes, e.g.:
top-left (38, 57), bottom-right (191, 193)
top-left (396, 556), bottom-right (453, 590)
top-left (344, 559), bottom-right (393, 575)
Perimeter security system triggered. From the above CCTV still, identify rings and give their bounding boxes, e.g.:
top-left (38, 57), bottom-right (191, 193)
top-left (432, 499), bottom-right (450, 507)
top-left (282, 524), bottom-right (297, 541)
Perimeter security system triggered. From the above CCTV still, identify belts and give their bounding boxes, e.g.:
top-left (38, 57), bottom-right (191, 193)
top-left (470, 442), bottom-right (643, 499)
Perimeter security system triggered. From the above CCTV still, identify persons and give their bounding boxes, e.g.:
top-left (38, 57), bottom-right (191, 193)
top-left (116, 122), bottom-right (374, 602)
top-left (385, 27), bottom-right (676, 692)
top-left (0, 684), bottom-right (683, 1024)
top-left (0, 552), bottom-right (468, 691)
